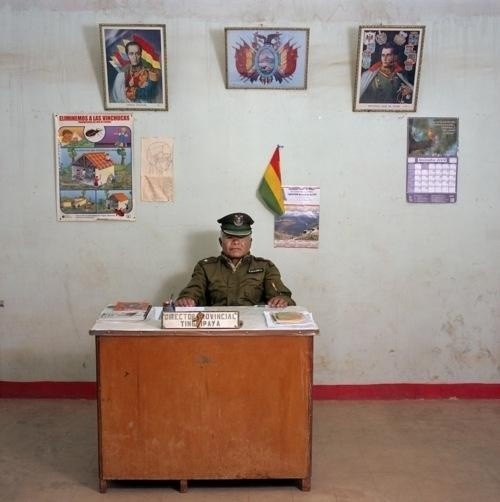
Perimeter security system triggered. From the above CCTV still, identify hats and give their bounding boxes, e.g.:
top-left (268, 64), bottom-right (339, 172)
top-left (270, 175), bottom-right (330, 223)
top-left (217, 213), bottom-right (254, 236)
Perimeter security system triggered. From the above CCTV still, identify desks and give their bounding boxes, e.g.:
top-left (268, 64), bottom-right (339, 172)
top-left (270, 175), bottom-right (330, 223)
top-left (89, 301), bottom-right (317, 495)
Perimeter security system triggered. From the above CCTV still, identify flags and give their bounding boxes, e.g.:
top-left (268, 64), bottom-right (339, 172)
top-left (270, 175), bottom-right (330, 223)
top-left (259, 147), bottom-right (284, 215)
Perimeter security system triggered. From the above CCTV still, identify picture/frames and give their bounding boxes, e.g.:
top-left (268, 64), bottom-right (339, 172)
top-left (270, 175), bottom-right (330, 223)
top-left (355, 22), bottom-right (426, 113)
top-left (99, 20), bottom-right (168, 113)
top-left (224, 25), bottom-right (309, 91)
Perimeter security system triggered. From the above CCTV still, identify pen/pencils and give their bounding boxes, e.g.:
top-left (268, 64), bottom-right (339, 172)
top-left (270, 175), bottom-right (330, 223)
top-left (168, 294), bottom-right (173, 305)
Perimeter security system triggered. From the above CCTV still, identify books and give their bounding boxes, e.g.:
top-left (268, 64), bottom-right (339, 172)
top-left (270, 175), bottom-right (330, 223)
top-left (271, 312), bottom-right (306, 323)
top-left (98, 303), bottom-right (153, 321)
top-left (114, 301), bottom-right (149, 314)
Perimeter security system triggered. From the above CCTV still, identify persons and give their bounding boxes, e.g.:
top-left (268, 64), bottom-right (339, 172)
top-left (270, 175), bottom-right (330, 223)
top-left (359, 41), bottom-right (413, 103)
top-left (176, 212), bottom-right (296, 308)
top-left (109, 42), bottom-right (162, 104)
